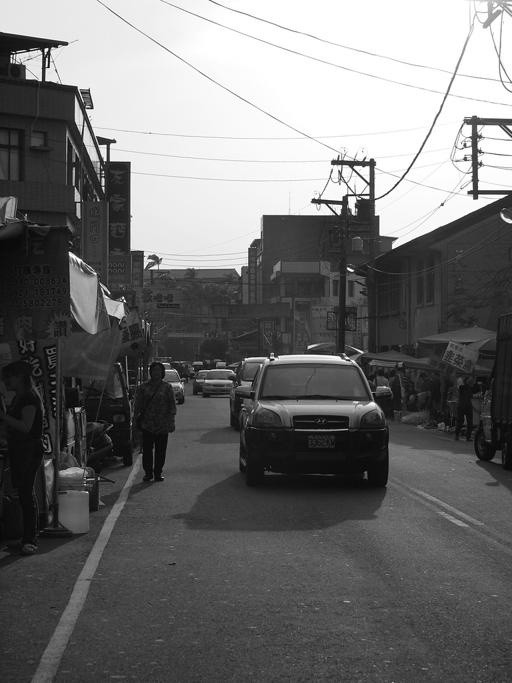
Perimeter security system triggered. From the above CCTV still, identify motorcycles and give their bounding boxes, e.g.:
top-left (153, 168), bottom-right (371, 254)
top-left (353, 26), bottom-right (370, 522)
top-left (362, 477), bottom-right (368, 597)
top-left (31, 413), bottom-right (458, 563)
top-left (61, 420), bottom-right (115, 474)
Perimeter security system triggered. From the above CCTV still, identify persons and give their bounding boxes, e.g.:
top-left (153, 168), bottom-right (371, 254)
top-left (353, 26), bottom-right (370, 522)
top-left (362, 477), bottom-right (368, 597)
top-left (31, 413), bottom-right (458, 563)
top-left (131, 361), bottom-right (177, 481)
top-left (173, 363), bottom-right (189, 383)
top-left (455, 376), bottom-right (480, 441)
top-left (364, 367), bottom-right (463, 427)
top-left (0, 360), bottom-right (45, 553)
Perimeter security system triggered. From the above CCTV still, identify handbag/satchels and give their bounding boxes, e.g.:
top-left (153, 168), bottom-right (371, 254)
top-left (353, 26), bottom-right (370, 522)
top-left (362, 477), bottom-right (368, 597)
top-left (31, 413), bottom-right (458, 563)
top-left (135, 415), bottom-right (147, 430)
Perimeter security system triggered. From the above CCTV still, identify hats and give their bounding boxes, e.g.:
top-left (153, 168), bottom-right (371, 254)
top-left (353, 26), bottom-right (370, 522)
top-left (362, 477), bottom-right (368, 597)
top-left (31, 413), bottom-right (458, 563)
top-left (3, 361), bottom-right (29, 384)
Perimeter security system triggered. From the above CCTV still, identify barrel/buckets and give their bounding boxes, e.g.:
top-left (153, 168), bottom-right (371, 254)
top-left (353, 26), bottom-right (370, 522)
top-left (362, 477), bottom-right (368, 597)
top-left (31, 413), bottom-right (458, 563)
top-left (56, 475), bottom-right (87, 491)
top-left (56, 491), bottom-right (89, 535)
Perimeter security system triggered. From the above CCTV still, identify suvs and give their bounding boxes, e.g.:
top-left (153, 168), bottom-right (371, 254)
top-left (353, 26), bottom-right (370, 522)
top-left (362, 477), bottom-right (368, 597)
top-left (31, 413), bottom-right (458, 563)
top-left (240, 352), bottom-right (392, 489)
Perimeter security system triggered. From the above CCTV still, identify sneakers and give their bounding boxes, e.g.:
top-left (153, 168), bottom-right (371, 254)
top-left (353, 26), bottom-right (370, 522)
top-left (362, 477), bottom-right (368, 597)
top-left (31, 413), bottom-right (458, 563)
top-left (143, 472), bottom-right (164, 481)
top-left (21, 540), bottom-right (40, 555)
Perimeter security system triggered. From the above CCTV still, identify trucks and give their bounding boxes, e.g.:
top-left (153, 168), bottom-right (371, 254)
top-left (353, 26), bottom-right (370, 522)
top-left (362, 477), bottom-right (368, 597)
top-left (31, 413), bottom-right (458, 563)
top-left (474, 313), bottom-right (512, 470)
top-left (83, 362), bottom-right (136, 466)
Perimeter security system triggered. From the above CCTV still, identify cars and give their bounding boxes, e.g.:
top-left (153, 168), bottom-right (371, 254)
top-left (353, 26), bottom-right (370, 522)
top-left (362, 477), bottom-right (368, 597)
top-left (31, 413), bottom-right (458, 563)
top-left (193, 369), bottom-right (236, 397)
top-left (163, 369), bottom-right (186, 404)
top-left (230, 356), bottom-right (267, 430)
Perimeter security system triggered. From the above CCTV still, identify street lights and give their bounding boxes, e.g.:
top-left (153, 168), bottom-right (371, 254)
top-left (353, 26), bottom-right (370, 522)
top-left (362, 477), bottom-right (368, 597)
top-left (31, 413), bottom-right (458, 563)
top-left (347, 264), bottom-right (377, 299)
